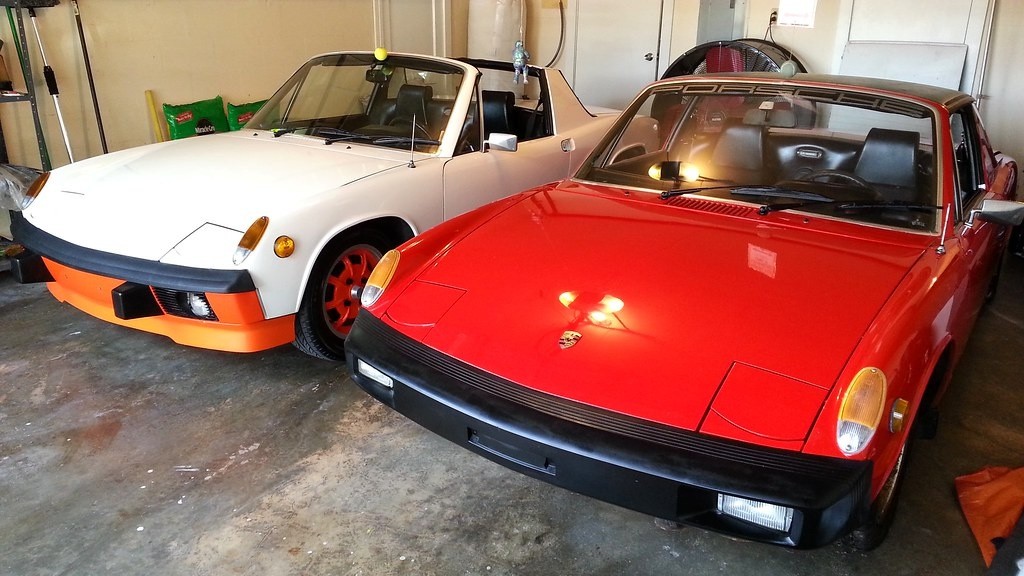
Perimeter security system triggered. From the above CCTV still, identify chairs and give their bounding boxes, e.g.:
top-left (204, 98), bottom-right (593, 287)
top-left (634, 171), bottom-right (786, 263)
top-left (361, 80), bottom-right (538, 161)
top-left (696, 117), bottom-right (937, 233)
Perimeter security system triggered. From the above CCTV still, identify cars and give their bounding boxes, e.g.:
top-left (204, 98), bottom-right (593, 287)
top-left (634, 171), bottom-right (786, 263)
top-left (9, 48), bottom-right (662, 365)
top-left (342, 68), bottom-right (1024, 556)
